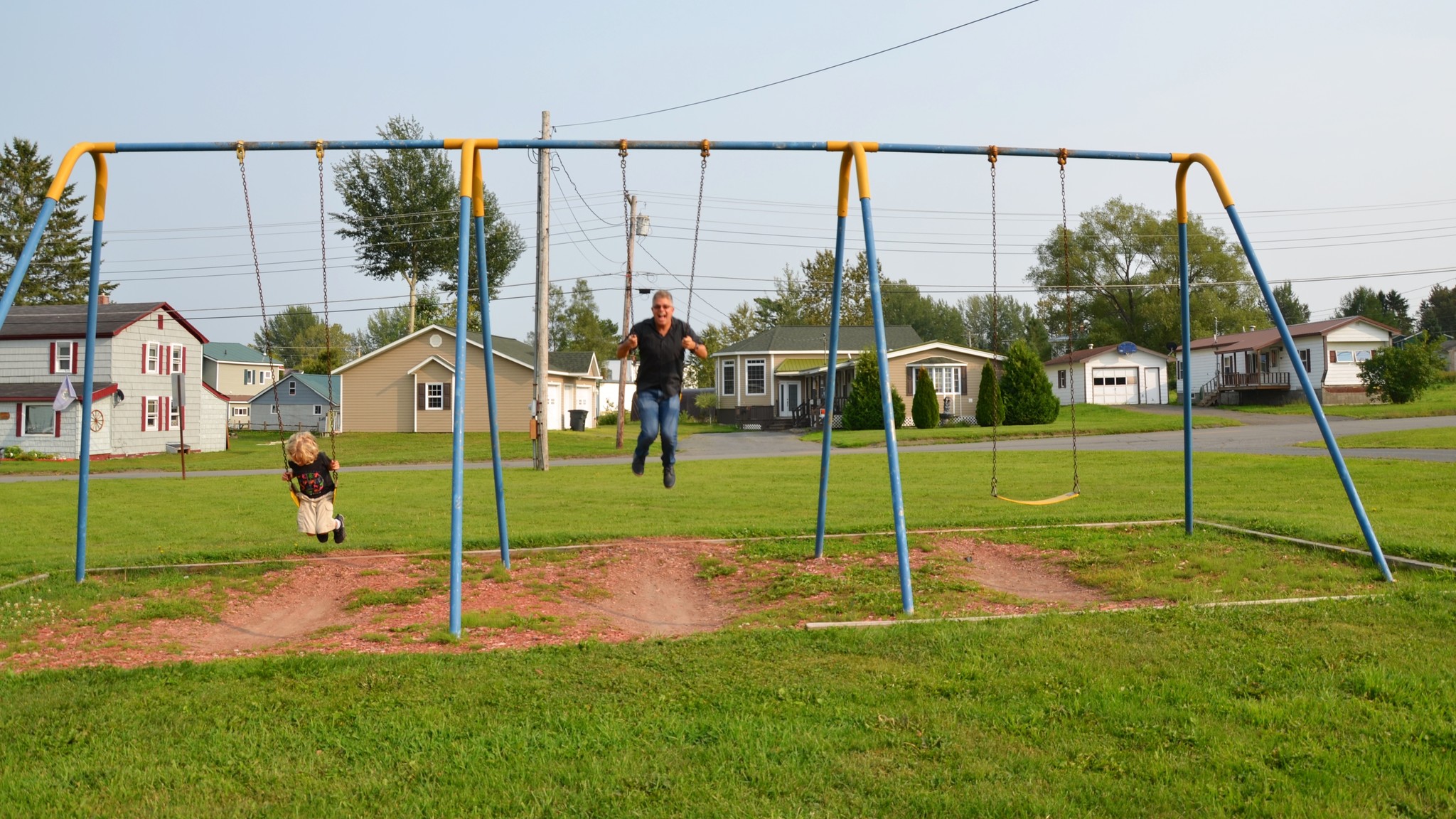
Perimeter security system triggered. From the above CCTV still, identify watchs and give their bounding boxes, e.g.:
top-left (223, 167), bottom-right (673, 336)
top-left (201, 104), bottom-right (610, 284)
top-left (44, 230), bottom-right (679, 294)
top-left (689, 343), bottom-right (698, 354)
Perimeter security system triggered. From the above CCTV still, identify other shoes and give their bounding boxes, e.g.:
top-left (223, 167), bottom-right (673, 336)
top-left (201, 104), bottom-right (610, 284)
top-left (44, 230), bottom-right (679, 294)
top-left (632, 451), bottom-right (645, 476)
top-left (663, 465), bottom-right (675, 488)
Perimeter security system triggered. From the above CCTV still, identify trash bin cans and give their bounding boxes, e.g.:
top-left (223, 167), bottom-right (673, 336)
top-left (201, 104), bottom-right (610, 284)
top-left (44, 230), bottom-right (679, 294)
top-left (568, 410), bottom-right (589, 432)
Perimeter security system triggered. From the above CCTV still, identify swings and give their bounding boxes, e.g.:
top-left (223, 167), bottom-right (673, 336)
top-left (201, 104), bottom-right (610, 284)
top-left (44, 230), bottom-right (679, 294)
top-left (987, 146), bottom-right (1080, 506)
top-left (618, 140), bottom-right (710, 398)
top-left (233, 142), bottom-right (339, 508)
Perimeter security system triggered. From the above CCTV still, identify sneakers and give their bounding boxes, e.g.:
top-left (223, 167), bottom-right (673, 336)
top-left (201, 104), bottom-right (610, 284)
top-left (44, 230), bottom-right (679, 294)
top-left (316, 532), bottom-right (329, 543)
top-left (333, 514), bottom-right (345, 543)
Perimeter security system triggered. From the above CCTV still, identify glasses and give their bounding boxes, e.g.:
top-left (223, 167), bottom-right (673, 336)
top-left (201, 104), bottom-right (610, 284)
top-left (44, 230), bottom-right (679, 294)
top-left (653, 303), bottom-right (672, 309)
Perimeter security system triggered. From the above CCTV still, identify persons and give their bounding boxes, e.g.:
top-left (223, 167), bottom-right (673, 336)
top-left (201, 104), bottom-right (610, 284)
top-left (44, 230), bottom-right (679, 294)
top-left (616, 290), bottom-right (708, 488)
top-left (281, 431), bottom-right (346, 544)
